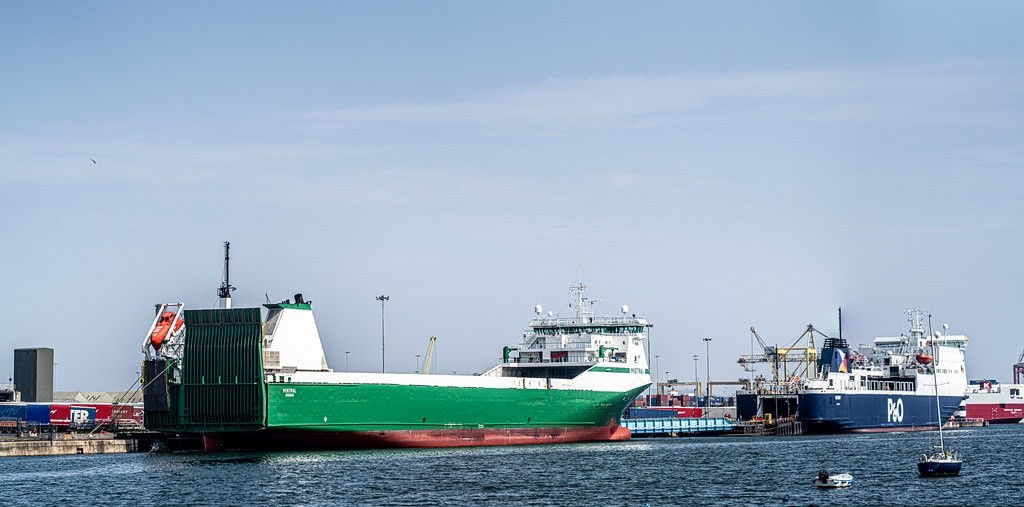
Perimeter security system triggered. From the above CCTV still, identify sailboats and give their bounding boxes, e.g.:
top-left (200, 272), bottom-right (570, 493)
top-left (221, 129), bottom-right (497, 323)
top-left (916, 312), bottom-right (962, 477)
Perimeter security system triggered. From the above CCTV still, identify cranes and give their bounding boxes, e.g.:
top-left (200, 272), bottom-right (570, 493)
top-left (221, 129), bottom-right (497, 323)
top-left (750, 325), bottom-right (792, 385)
top-left (421, 335), bottom-right (436, 375)
top-left (736, 323), bottom-right (819, 379)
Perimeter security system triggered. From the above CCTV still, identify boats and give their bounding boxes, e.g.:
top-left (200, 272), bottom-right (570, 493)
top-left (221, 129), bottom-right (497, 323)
top-left (815, 473), bottom-right (853, 488)
top-left (136, 240), bottom-right (654, 450)
top-left (793, 307), bottom-right (970, 435)
